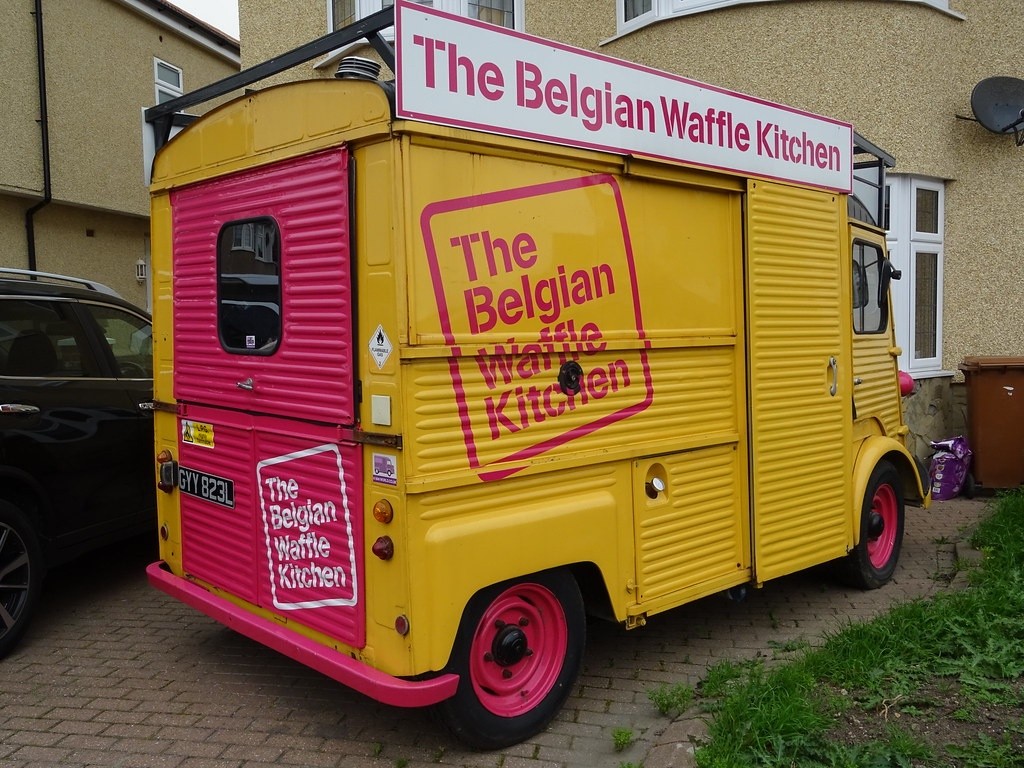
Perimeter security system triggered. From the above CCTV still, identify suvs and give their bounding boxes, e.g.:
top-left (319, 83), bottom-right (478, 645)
top-left (0, 263), bottom-right (152, 653)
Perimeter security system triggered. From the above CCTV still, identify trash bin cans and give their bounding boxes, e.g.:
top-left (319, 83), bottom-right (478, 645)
top-left (957, 355), bottom-right (1024, 502)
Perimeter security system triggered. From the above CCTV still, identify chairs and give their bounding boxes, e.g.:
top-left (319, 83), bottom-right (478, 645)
top-left (6, 328), bottom-right (60, 378)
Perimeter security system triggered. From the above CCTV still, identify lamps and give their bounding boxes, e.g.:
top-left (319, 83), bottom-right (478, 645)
top-left (136, 257), bottom-right (147, 285)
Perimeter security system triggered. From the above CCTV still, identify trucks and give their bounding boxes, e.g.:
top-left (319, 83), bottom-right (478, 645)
top-left (141, 0), bottom-right (933, 757)
